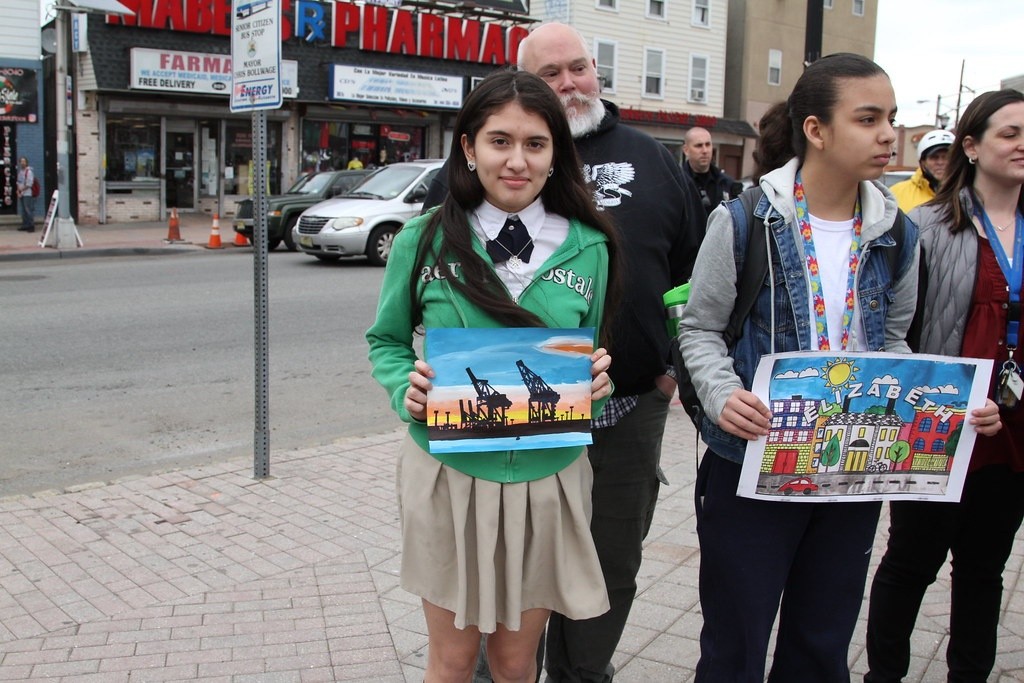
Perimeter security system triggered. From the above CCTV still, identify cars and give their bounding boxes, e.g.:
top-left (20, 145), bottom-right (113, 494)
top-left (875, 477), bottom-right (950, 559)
top-left (233, 169), bottom-right (376, 251)
top-left (291, 159), bottom-right (452, 266)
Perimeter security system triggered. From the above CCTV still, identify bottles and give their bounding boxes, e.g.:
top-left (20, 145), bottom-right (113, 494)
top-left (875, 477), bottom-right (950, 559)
top-left (663, 281), bottom-right (691, 338)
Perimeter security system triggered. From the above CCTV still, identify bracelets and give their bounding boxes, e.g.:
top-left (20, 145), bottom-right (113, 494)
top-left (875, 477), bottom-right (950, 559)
top-left (666, 367), bottom-right (678, 381)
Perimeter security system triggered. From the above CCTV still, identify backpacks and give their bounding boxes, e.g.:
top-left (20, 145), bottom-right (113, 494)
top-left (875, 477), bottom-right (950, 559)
top-left (31, 177), bottom-right (41, 197)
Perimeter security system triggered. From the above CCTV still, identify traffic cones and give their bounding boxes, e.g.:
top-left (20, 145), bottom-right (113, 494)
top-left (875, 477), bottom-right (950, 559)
top-left (164, 208), bottom-right (185, 241)
top-left (232, 233), bottom-right (251, 246)
top-left (204, 214), bottom-right (224, 249)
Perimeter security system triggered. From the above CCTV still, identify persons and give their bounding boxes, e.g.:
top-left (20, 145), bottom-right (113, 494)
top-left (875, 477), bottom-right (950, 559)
top-left (365, 72), bottom-right (615, 683)
top-left (865, 89), bottom-right (1024, 683)
top-left (679, 50), bottom-right (1002, 683)
top-left (374, 149), bottom-right (387, 166)
top-left (682, 127), bottom-right (743, 214)
top-left (16, 157), bottom-right (35, 231)
top-left (472, 24), bottom-right (693, 683)
top-left (889, 130), bottom-right (955, 214)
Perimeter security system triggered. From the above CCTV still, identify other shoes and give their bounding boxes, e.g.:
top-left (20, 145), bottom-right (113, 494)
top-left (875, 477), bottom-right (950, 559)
top-left (18, 225), bottom-right (26, 231)
top-left (26, 227), bottom-right (35, 231)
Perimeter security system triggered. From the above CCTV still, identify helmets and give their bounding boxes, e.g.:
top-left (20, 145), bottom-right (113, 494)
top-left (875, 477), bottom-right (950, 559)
top-left (920, 131), bottom-right (956, 186)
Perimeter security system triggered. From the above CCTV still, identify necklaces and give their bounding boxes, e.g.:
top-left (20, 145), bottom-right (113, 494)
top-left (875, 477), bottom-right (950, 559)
top-left (495, 239), bottom-right (532, 267)
top-left (994, 220), bottom-right (1015, 231)
